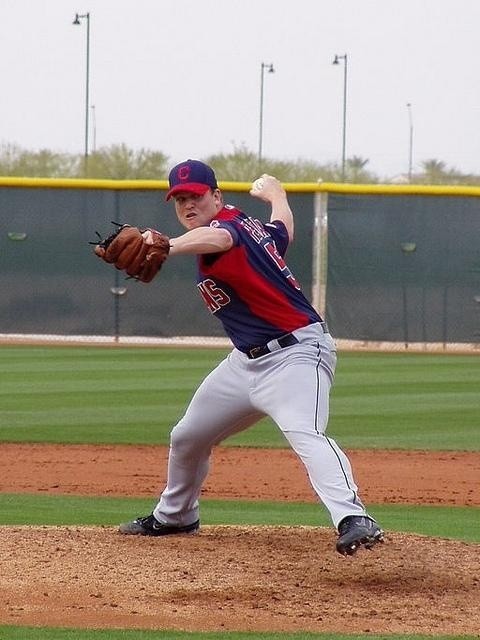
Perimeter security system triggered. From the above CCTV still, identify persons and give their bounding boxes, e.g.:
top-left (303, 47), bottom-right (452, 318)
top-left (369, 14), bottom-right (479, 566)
top-left (88, 155), bottom-right (390, 561)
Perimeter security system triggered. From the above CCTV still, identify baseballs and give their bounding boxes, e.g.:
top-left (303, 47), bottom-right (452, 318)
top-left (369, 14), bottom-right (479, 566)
top-left (253, 178), bottom-right (262, 190)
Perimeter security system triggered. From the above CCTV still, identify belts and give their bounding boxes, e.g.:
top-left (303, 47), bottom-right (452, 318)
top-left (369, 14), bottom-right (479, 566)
top-left (246, 322), bottom-right (328, 359)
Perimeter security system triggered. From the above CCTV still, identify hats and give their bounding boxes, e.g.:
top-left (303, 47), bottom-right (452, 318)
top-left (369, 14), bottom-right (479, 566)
top-left (166, 159), bottom-right (219, 203)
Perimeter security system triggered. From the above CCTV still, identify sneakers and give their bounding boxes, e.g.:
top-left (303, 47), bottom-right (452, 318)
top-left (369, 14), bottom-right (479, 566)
top-left (119, 511), bottom-right (201, 537)
top-left (335, 515), bottom-right (385, 558)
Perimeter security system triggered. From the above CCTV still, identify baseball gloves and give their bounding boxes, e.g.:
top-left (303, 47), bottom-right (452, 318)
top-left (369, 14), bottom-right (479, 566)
top-left (87, 220), bottom-right (171, 284)
